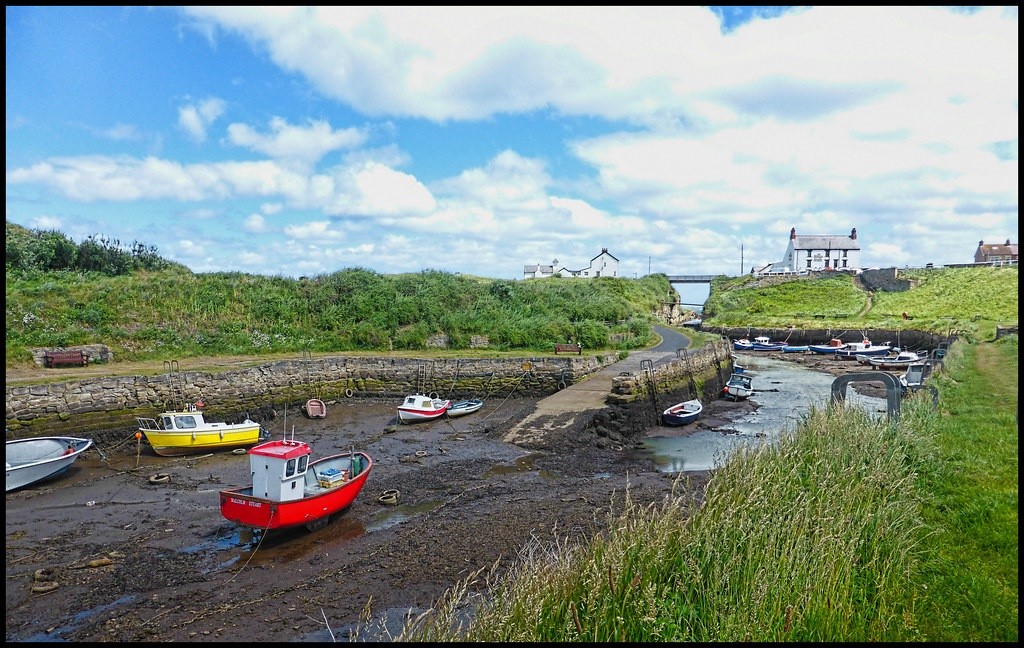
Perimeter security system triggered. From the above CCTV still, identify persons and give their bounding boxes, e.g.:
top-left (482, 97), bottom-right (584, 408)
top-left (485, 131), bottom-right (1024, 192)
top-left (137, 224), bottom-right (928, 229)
top-left (389, 359), bottom-right (396, 375)
top-left (344, 358), bottom-right (357, 369)
top-left (61, 445), bottom-right (75, 456)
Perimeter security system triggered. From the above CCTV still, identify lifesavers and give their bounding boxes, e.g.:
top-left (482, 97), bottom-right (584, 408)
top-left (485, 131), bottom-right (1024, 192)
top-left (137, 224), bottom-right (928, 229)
top-left (32, 581), bottom-right (60, 593)
top-left (233, 448), bottom-right (246, 454)
top-left (379, 495), bottom-right (396, 505)
top-left (557, 382), bottom-right (567, 391)
top-left (415, 451), bottom-right (427, 457)
top-left (346, 388), bottom-right (353, 398)
top-left (610, 445), bottom-right (622, 451)
top-left (384, 425), bottom-right (396, 433)
top-left (33, 568), bottom-right (59, 580)
top-left (429, 392), bottom-right (439, 399)
top-left (382, 488), bottom-right (400, 498)
top-left (150, 473), bottom-right (170, 484)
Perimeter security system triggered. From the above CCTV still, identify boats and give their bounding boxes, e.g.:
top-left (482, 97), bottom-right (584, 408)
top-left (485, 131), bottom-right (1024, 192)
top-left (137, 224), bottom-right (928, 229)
top-left (447, 399), bottom-right (483, 419)
top-left (810, 338), bottom-right (871, 354)
top-left (835, 342), bottom-right (891, 358)
top-left (662, 399), bottom-right (702, 426)
top-left (734, 338), bottom-right (753, 351)
top-left (752, 337), bottom-right (789, 351)
top-left (782, 345), bottom-right (810, 352)
top-left (725, 368), bottom-right (753, 400)
top-left (869, 352), bottom-right (920, 367)
top-left (218, 401), bottom-right (373, 530)
top-left (899, 363), bottom-right (934, 397)
top-left (855, 347), bottom-right (901, 365)
top-left (396, 394), bottom-right (450, 424)
top-left (5, 436), bottom-right (93, 494)
top-left (137, 360), bottom-right (261, 456)
